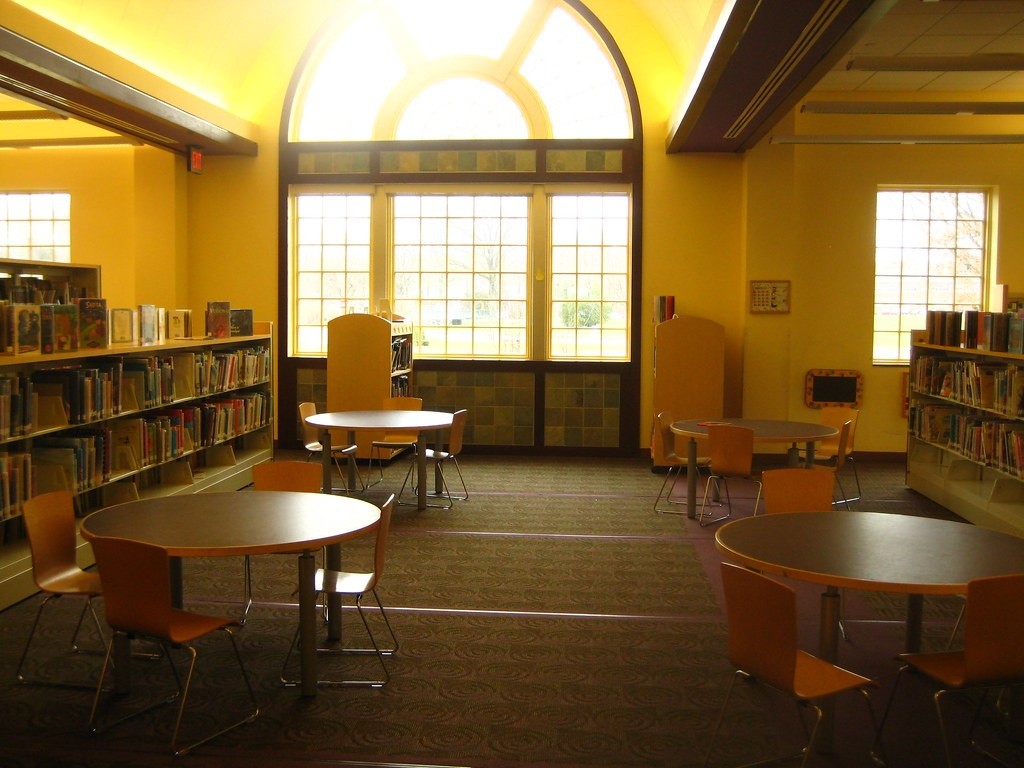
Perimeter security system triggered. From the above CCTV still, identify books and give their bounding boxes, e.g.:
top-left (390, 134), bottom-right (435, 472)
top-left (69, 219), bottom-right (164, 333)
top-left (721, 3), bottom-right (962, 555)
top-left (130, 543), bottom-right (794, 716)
top-left (912, 353), bottom-right (1024, 419)
top-left (1, 345), bottom-right (273, 442)
top-left (926, 311), bottom-right (1024, 355)
top-left (909, 399), bottom-right (1024, 479)
top-left (112, 307), bottom-right (133, 343)
top-left (392, 374), bottom-right (409, 398)
top-left (230, 309), bottom-right (252, 337)
top-left (9, 303), bottom-right (41, 353)
top-left (42, 303), bottom-right (79, 353)
top-left (205, 301), bottom-right (231, 338)
top-left (71, 297), bottom-right (109, 348)
top-left (391, 336), bottom-right (411, 372)
top-left (0, 389), bottom-right (274, 514)
top-left (140, 304), bottom-right (159, 343)
top-left (159, 307), bottom-right (167, 343)
top-left (0, 276), bottom-right (87, 307)
top-left (654, 296), bottom-right (674, 324)
top-left (168, 310), bottom-right (193, 339)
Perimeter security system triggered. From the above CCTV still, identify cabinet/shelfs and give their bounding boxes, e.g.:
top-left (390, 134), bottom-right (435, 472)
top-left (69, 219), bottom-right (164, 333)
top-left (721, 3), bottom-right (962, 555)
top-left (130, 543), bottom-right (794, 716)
top-left (325, 311), bottom-right (415, 459)
top-left (904, 282), bottom-right (1024, 539)
top-left (650, 317), bottom-right (725, 475)
top-left (0, 256), bottom-right (275, 611)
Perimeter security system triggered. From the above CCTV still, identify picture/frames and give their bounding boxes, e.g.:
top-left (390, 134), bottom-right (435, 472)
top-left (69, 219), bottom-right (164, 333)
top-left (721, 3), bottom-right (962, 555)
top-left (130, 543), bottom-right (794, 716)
top-left (750, 279), bottom-right (791, 313)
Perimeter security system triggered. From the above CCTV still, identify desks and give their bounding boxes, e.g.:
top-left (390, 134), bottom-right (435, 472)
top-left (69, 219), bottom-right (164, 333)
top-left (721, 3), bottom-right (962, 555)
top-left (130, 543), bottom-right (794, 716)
top-left (669, 419), bottom-right (840, 519)
top-left (715, 511), bottom-right (1024, 756)
top-left (305, 410), bottom-right (455, 510)
top-left (79, 492), bottom-right (383, 695)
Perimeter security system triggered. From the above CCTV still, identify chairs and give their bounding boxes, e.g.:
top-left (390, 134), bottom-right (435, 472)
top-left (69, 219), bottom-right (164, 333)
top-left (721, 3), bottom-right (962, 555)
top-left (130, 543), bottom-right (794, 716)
top-left (814, 419), bottom-right (850, 512)
top-left (869, 573), bottom-right (1024, 768)
top-left (815, 406), bottom-right (861, 505)
top-left (299, 403), bottom-right (365, 496)
top-left (397, 409), bottom-right (469, 509)
top-left (280, 492), bottom-right (401, 687)
top-left (760, 467), bottom-right (849, 641)
top-left (654, 411), bottom-right (724, 516)
top-left (15, 491), bottom-right (117, 694)
top-left (367, 397), bottom-right (422, 489)
top-left (87, 539), bottom-right (260, 757)
top-left (239, 461), bottom-right (332, 625)
top-left (699, 425), bottom-right (764, 528)
top-left (706, 562), bottom-right (890, 768)
